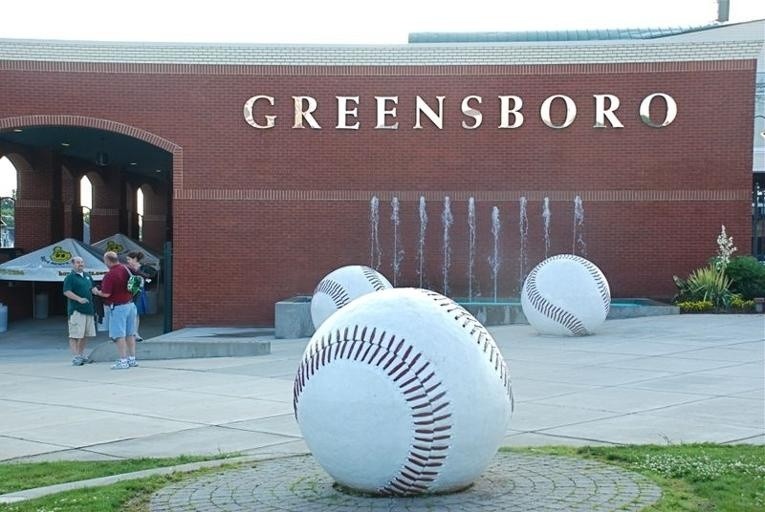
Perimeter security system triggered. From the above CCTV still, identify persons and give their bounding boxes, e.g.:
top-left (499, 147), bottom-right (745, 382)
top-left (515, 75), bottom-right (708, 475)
top-left (126, 251), bottom-right (149, 342)
top-left (90, 249), bottom-right (137, 370)
top-left (62, 256), bottom-right (98, 367)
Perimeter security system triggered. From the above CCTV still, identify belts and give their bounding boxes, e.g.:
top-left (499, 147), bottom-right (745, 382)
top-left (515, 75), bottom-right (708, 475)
top-left (114, 300), bottom-right (134, 306)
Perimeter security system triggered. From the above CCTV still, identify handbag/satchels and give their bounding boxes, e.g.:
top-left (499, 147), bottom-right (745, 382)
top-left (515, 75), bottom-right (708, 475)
top-left (123, 264), bottom-right (144, 295)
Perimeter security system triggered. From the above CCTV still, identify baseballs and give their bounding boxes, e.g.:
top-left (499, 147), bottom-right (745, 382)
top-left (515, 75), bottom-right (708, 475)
top-left (310, 265), bottom-right (392, 332)
top-left (520, 255), bottom-right (611, 337)
top-left (291, 287), bottom-right (516, 497)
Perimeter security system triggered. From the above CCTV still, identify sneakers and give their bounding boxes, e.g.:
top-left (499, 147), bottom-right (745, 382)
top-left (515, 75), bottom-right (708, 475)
top-left (72, 355), bottom-right (94, 365)
top-left (110, 357), bottom-right (138, 369)
top-left (137, 333), bottom-right (144, 341)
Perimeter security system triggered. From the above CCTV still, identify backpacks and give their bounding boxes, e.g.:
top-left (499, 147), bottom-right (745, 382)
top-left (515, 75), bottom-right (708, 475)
top-left (138, 262), bottom-right (158, 290)
